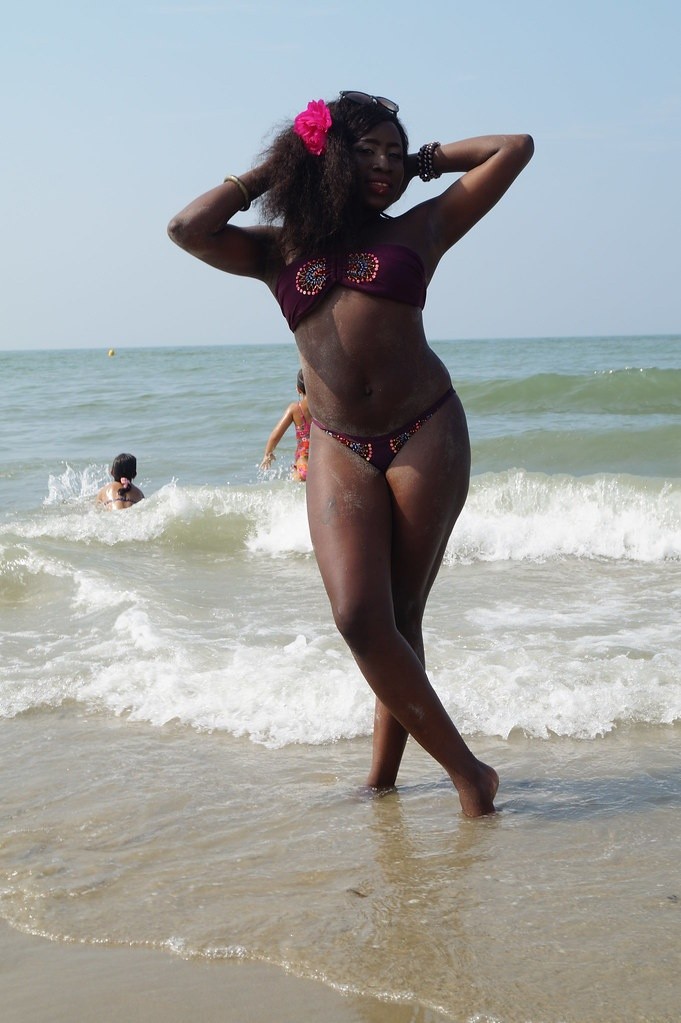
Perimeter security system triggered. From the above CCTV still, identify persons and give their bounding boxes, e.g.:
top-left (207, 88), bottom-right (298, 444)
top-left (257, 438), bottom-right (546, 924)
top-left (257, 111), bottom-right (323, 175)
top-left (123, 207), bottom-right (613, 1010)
top-left (168, 91), bottom-right (535, 814)
top-left (259, 369), bottom-right (313, 481)
top-left (97, 453), bottom-right (144, 511)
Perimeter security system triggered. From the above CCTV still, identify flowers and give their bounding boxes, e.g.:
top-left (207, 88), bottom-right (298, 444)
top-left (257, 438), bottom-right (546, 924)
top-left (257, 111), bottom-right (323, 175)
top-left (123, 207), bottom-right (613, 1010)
top-left (292, 99), bottom-right (332, 156)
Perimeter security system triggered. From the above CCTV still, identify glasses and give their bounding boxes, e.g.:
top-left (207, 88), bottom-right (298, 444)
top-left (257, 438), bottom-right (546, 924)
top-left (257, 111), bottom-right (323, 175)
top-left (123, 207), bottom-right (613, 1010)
top-left (336, 91), bottom-right (400, 117)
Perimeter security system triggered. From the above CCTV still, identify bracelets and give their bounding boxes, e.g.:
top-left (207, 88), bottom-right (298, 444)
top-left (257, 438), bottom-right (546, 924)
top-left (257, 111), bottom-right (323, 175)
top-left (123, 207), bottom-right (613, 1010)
top-left (224, 175), bottom-right (250, 212)
top-left (417, 141), bottom-right (442, 182)
